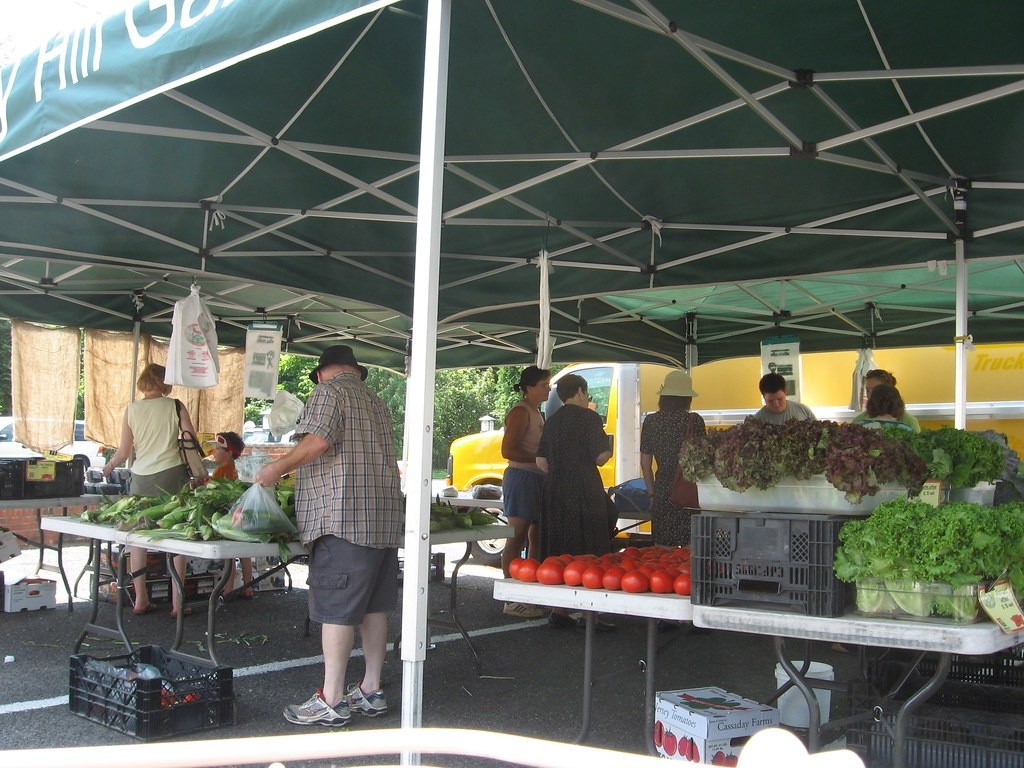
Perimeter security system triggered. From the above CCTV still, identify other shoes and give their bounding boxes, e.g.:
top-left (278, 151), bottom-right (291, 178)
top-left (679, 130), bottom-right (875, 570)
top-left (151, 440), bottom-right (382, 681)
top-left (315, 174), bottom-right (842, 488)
top-left (658, 620), bottom-right (682, 632)
top-left (547, 613), bottom-right (579, 628)
top-left (576, 616), bottom-right (616, 631)
top-left (239, 589), bottom-right (253, 599)
top-left (220, 591), bottom-right (233, 602)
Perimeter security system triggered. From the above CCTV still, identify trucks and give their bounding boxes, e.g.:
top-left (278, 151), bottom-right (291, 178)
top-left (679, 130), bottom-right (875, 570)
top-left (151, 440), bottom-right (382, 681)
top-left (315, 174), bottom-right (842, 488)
top-left (444, 340), bottom-right (1023, 567)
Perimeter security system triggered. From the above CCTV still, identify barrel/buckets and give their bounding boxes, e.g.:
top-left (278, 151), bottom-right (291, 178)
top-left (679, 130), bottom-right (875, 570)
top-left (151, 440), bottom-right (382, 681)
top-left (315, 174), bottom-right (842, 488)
top-left (774, 661), bottom-right (834, 728)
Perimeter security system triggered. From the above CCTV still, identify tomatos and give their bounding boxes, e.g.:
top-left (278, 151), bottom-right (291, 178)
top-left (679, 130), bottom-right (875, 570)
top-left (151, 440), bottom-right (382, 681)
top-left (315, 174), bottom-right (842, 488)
top-left (509, 545), bottom-right (692, 596)
top-left (160, 688), bottom-right (201, 707)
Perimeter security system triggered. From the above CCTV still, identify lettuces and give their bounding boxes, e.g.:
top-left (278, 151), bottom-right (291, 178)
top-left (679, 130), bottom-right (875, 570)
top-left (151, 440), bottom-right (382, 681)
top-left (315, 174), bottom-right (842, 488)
top-left (831, 494), bottom-right (1024, 622)
top-left (678, 414), bottom-right (1008, 504)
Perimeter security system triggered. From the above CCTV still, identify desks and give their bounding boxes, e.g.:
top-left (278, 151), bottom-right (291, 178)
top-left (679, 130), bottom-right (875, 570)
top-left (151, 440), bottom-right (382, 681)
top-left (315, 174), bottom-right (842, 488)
top-left (491, 545), bottom-right (1024, 768)
top-left (432, 487), bottom-right (653, 537)
top-left (41, 515), bottom-right (316, 662)
top-left (108, 525), bottom-right (515, 729)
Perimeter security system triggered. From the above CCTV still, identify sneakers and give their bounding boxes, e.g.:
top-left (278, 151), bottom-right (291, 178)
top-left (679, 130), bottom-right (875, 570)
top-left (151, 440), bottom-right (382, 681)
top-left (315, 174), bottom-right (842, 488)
top-left (504, 601), bottom-right (543, 617)
top-left (344, 682), bottom-right (388, 718)
top-left (283, 688), bottom-right (351, 726)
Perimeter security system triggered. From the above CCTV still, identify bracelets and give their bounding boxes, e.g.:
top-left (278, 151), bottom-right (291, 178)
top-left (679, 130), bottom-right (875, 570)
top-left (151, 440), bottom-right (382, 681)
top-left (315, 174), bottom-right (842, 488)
top-left (647, 493), bottom-right (654, 498)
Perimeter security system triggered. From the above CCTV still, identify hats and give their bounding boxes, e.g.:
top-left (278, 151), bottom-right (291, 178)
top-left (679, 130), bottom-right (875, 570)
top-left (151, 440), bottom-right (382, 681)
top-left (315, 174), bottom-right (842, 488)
top-left (655, 370), bottom-right (697, 396)
top-left (310, 346), bottom-right (368, 384)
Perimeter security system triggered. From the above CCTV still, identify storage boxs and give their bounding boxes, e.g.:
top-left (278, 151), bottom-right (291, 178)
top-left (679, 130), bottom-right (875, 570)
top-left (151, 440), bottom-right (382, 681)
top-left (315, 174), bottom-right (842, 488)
top-left (656, 686), bottom-right (780, 768)
top-left (854, 579), bottom-right (993, 627)
top-left (5, 578), bottom-right (57, 612)
top-left (68, 644), bottom-right (235, 742)
top-left (90, 543), bottom-right (285, 605)
top-left (694, 466), bottom-right (908, 516)
top-left (846, 643), bottom-right (1024, 768)
top-left (0, 450), bottom-right (25, 500)
top-left (0, 530), bottom-right (21, 564)
top-left (22, 458), bottom-right (84, 500)
top-left (684, 506), bottom-right (863, 617)
top-left (397, 552), bottom-right (445, 586)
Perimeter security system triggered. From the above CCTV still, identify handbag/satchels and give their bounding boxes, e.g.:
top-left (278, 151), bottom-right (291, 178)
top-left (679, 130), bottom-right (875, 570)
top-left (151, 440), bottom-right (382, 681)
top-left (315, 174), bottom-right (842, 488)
top-left (670, 412), bottom-right (698, 508)
top-left (174, 397), bottom-right (208, 481)
top-left (230, 449), bottom-right (297, 535)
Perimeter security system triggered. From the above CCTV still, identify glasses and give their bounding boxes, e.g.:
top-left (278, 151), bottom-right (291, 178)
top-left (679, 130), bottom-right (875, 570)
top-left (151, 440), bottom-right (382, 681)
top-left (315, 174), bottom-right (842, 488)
top-left (216, 436), bottom-right (228, 448)
top-left (581, 391), bottom-right (593, 401)
top-left (534, 382), bottom-right (549, 389)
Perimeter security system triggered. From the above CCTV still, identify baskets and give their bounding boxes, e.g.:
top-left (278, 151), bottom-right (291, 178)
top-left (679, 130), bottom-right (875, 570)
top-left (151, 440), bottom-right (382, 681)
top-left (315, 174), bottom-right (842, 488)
top-left (69, 644), bottom-right (233, 742)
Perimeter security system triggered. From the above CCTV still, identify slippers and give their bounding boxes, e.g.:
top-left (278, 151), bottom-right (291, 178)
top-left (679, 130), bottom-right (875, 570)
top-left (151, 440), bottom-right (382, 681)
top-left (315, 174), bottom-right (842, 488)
top-left (170, 606), bottom-right (192, 617)
top-left (133, 602), bottom-right (157, 615)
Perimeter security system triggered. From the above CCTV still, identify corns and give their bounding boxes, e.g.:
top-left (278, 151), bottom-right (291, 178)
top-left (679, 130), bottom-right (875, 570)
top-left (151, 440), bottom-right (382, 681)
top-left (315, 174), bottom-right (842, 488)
top-left (80, 476), bottom-right (501, 545)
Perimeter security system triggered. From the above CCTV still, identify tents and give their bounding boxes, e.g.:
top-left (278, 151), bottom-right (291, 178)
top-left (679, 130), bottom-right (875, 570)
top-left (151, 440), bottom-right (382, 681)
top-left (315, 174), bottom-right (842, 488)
top-left (1, 0), bottom-right (1024, 768)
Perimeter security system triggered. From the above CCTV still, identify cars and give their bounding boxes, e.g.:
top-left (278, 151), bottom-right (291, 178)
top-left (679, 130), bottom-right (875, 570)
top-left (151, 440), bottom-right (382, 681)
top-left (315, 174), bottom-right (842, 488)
top-left (0, 416), bottom-right (85, 445)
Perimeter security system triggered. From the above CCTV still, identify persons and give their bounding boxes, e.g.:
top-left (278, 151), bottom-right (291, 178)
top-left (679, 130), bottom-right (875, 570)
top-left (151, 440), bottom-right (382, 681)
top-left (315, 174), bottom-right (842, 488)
top-left (850, 369), bottom-right (921, 436)
top-left (257, 345), bottom-right (405, 727)
top-left (102, 364), bottom-right (208, 618)
top-left (857, 385), bottom-right (916, 435)
top-left (750, 373), bottom-right (819, 427)
top-left (501, 364), bottom-right (549, 618)
top-left (213, 432), bottom-right (255, 603)
top-left (639, 370), bottom-right (707, 551)
top-left (537, 373), bottom-right (620, 631)
